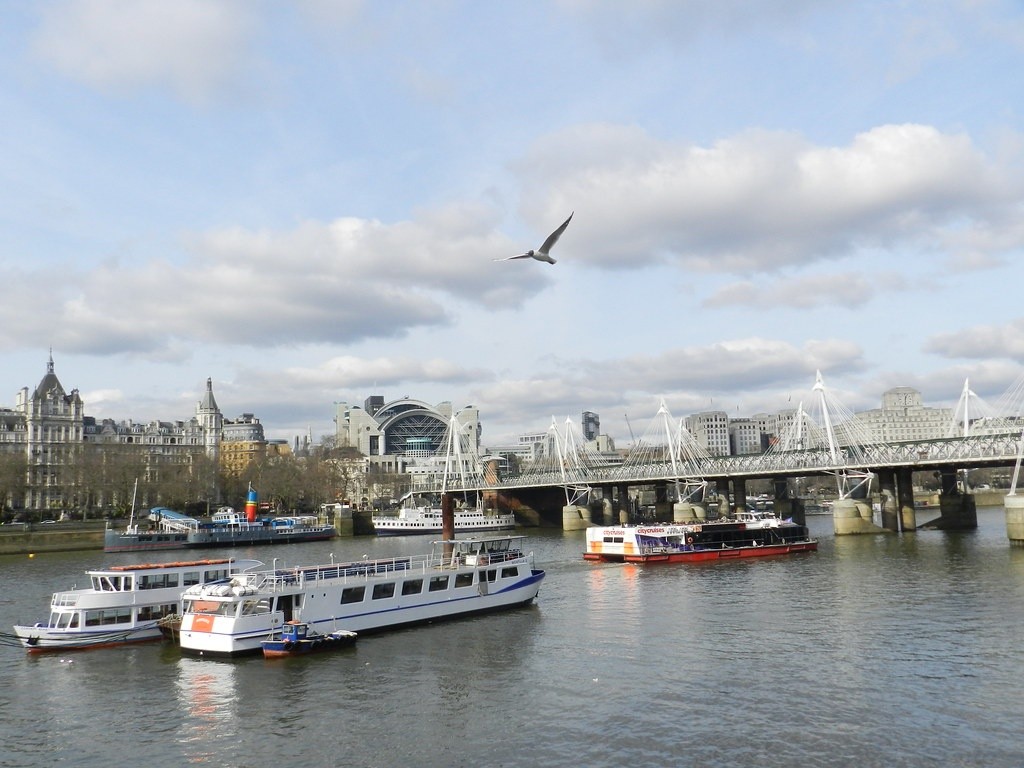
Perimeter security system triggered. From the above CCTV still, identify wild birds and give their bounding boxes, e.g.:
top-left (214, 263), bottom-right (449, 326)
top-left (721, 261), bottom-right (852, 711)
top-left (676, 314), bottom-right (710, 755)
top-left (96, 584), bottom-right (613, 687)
top-left (491, 209), bottom-right (576, 266)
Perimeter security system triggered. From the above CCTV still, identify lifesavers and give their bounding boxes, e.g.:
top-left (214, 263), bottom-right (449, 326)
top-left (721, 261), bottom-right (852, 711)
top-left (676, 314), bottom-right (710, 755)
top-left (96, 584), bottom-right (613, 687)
top-left (311, 637), bottom-right (356, 651)
top-left (283, 641), bottom-right (303, 651)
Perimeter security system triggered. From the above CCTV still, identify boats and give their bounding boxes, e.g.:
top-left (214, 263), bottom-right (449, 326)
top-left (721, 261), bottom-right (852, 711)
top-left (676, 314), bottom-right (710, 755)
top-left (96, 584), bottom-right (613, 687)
top-left (371, 489), bottom-right (517, 538)
top-left (581, 478), bottom-right (819, 565)
top-left (102, 477), bottom-right (338, 555)
top-left (260, 619), bottom-right (358, 660)
top-left (180, 494), bottom-right (547, 659)
top-left (12, 557), bottom-right (264, 655)
